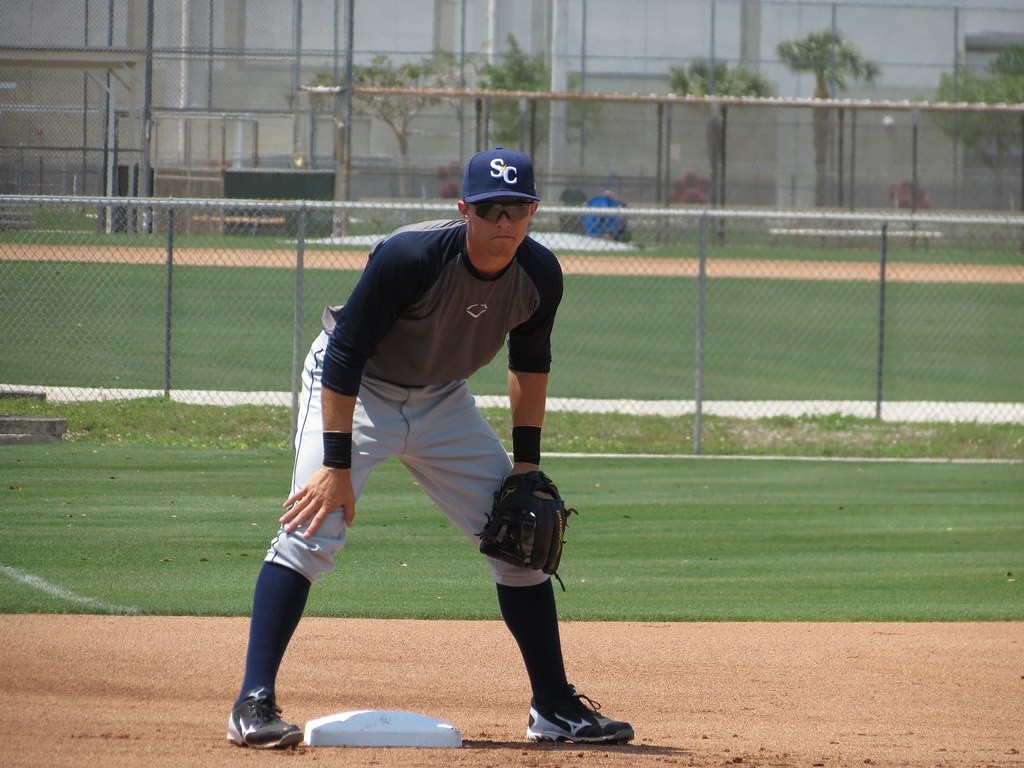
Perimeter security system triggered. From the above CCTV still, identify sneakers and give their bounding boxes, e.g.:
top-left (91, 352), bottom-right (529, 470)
top-left (227, 686), bottom-right (304, 749)
top-left (528, 684), bottom-right (634, 743)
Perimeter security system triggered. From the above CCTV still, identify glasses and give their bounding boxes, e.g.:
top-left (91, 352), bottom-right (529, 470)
top-left (471, 200), bottom-right (533, 220)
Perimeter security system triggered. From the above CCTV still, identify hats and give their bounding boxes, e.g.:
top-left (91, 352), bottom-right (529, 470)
top-left (461, 147), bottom-right (540, 204)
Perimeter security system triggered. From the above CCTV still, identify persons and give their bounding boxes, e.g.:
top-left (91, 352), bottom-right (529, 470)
top-left (226, 148), bottom-right (635, 749)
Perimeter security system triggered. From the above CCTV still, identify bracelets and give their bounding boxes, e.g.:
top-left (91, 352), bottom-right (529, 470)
top-left (322, 429), bottom-right (352, 470)
top-left (511, 425), bottom-right (542, 465)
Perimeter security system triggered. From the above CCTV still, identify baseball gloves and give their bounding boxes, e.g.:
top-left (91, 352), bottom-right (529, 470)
top-left (473, 470), bottom-right (579, 592)
